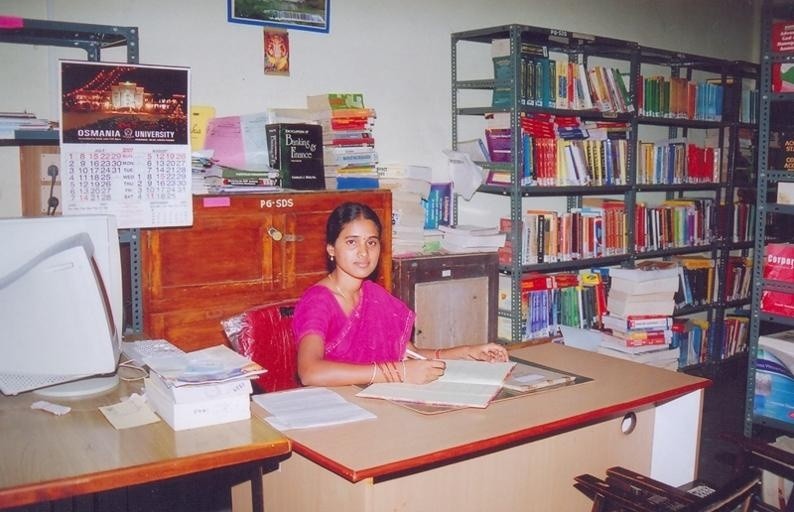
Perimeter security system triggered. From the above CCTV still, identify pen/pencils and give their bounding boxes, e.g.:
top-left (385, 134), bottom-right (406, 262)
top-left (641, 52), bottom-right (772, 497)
top-left (405, 346), bottom-right (427, 361)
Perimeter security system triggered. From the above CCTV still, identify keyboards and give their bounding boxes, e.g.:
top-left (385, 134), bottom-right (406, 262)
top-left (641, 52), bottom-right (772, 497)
top-left (133, 339), bottom-right (186, 358)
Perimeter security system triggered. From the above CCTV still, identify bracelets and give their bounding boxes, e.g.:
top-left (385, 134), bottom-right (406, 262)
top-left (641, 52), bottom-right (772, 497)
top-left (433, 348), bottom-right (447, 358)
top-left (362, 362), bottom-right (409, 385)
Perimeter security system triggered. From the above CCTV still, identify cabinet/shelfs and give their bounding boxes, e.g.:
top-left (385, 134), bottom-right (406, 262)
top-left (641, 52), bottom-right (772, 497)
top-left (144, 187), bottom-right (395, 359)
top-left (1, 13), bottom-right (145, 340)
top-left (742, 0), bottom-right (792, 448)
top-left (393, 256), bottom-right (501, 349)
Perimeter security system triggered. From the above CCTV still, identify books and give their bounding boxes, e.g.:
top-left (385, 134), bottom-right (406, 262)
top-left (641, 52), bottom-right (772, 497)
top-left (498, 362), bottom-right (577, 394)
top-left (137, 343), bottom-right (268, 432)
top-left (2, 110), bottom-right (54, 132)
top-left (377, 163), bottom-right (506, 256)
top-left (492, 39), bottom-right (750, 124)
top-left (486, 112), bottom-right (751, 371)
top-left (755, 17), bottom-right (794, 509)
top-left (189, 93), bottom-right (389, 195)
top-left (359, 358), bottom-right (518, 410)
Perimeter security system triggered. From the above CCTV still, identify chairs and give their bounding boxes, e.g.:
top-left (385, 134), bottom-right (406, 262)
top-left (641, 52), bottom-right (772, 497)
top-left (224, 297), bottom-right (308, 392)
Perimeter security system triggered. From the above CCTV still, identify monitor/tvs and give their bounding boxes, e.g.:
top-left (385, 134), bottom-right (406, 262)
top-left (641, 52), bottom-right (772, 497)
top-left (0, 231), bottom-right (121, 398)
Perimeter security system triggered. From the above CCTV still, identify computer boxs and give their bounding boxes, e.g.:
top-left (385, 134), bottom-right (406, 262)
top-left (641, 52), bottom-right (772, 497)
top-left (0, 214), bottom-right (123, 353)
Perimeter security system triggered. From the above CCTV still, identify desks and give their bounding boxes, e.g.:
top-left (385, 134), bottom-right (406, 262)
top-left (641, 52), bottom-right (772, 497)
top-left (0, 340), bottom-right (293, 512)
top-left (250, 336), bottom-right (719, 512)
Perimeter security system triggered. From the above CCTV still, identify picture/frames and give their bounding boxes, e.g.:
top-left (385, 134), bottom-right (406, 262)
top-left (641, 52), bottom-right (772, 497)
top-left (226, 0), bottom-right (331, 36)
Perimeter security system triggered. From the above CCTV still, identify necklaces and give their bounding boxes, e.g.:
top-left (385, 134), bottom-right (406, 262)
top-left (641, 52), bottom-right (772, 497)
top-left (326, 273), bottom-right (364, 321)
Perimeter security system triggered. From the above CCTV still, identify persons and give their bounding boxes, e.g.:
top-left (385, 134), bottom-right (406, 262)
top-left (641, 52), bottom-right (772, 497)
top-left (291, 200), bottom-right (515, 386)
